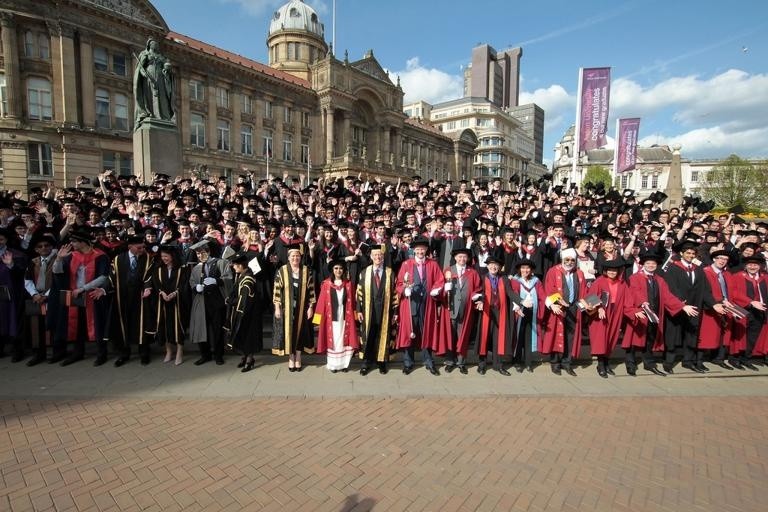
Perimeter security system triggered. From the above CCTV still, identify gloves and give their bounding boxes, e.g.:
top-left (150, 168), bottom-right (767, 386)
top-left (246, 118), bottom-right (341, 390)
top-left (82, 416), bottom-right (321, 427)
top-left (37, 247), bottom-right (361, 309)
top-left (444, 282), bottom-right (453, 293)
top-left (472, 294), bottom-right (483, 302)
top-left (430, 287), bottom-right (442, 297)
top-left (196, 284), bottom-right (205, 292)
top-left (204, 277), bottom-right (217, 286)
top-left (405, 287), bottom-right (412, 297)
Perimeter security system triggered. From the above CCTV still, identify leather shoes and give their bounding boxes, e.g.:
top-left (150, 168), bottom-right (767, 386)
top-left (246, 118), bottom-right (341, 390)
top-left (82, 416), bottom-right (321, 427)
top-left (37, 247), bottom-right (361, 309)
top-left (331, 362), bottom-right (576, 376)
top-left (289, 367), bottom-right (302, 372)
top-left (11, 350), bottom-right (225, 367)
top-left (597, 359), bottom-right (759, 378)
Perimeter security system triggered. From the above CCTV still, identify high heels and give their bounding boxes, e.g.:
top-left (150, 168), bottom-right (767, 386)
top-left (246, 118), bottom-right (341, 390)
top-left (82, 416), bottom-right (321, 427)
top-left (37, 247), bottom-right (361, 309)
top-left (238, 357), bottom-right (255, 372)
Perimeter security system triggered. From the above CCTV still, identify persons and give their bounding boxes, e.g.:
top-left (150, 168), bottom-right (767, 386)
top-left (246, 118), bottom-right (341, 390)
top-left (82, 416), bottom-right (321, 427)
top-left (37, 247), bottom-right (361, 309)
top-left (0, 174), bottom-right (768, 377)
top-left (132, 38), bottom-right (174, 119)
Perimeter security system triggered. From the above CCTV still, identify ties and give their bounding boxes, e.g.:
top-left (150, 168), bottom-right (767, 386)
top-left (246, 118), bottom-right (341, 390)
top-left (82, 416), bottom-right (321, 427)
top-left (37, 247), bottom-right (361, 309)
top-left (375, 270), bottom-right (381, 289)
top-left (647, 275), bottom-right (654, 289)
top-left (418, 261), bottom-right (424, 284)
top-left (493, 277), bottom-right (497, 291)
top-left (688, 266), bottom-right (692, 284)
top-left (719, 274), bottom-right (727, 299)
top-left (566, 273), bottom-right (574, 304)
top-left (459, 267), bottom-right (463, 285)
